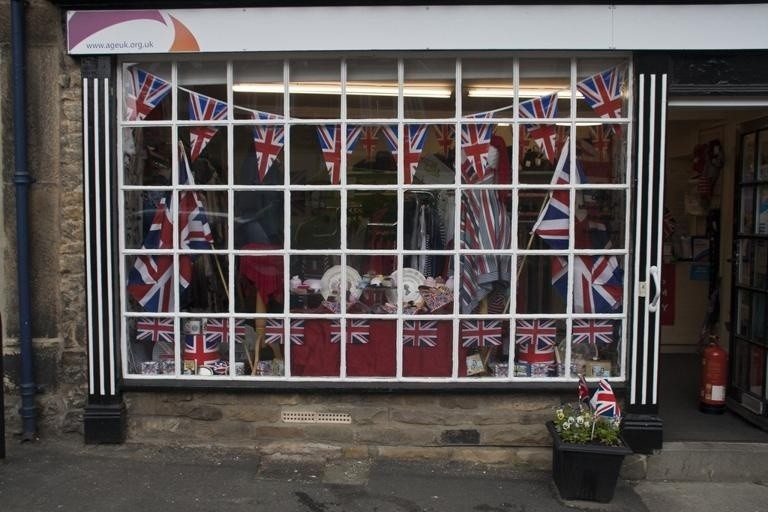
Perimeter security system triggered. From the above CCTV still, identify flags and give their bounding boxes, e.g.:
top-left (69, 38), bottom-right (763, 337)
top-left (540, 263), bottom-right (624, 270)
top-left (460, 319), bottom-right (504, 348)
top-left (572, 319), bottom-right (615, 344)
top-left (329, 318), bottom-right (370, 345)
top-left (591, 377), bottom-right (622, 418)
top-left (402, 320), bottom-right (439, 348)
top-left (129, 142), bottom-right (215, 313)
top-left (264, 318), bottom-right (304, 346)
top-left (515, 318), bottom-right (557, 345)
top-left (206, 317), bottom-right (246, 344)
top-left (577, 377), bottom-right (589, 411)
top-left (135, 317), bottom-right (173, 341)
top-left (533, 137), bottom-right (623, 312)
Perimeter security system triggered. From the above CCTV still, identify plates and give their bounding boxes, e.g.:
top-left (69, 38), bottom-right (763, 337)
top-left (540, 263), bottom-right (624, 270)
top-left (384, 268), bottom-right (427, 309)
top-left (318, 264), bottom-right (364, 305)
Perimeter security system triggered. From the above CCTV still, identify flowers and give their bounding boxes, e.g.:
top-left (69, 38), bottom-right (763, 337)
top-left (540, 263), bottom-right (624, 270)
top-left (552, 403), bottom-right (622, 445)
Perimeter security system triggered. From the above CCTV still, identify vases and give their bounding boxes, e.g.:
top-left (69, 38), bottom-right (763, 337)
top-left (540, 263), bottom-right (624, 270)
top-left (545, 420), bottom-right (632, 504)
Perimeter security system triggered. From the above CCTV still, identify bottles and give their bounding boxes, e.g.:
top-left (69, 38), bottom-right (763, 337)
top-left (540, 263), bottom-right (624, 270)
top-left (290, 275), bottom-right (302, 292)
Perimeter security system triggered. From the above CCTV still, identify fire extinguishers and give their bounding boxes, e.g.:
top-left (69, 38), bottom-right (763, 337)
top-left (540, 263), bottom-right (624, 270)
top-left (698, 334), bottom-right (729, 415)
